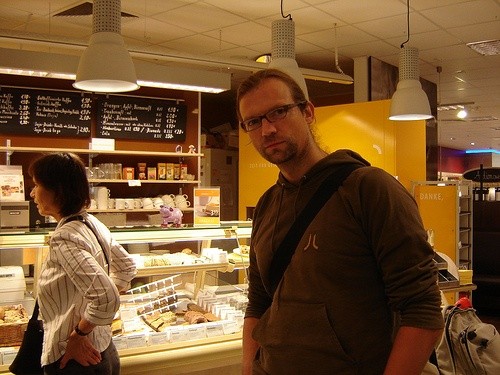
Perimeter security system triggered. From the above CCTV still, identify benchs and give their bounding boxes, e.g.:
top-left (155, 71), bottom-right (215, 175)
top-left (474, 274), bottom-right (500, 287)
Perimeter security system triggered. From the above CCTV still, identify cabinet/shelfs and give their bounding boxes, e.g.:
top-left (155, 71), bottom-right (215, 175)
top-left (412, 181), bottom-right (474, 310)
top-left (0, 223), bottom-right (460, 375)
top-left (0, 140), bottom-right (204, 284)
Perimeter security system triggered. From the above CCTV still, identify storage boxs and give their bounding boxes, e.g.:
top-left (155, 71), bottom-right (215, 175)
top-left (180, 164), bottom-right (187, 180)
top-left (166, 163), bottom-right (174, 180)
top-left (201, 134), bottom-right (207, 147)
top-left (229, 128), bottom-right (239, 147)
top-left (147, 167), bottom-right (156, 180)
top-left (174, 164), bottom-right (180, 179)
top-left (157, 163), bottom-right (166, 180)
top-left (123, 167), bottom-right (135, 180)
top-left (138, 163), bottom-right (147, 180)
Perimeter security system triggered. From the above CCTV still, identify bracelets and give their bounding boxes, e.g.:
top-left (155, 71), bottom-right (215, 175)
top-left (74, 323), bottom-right (92, 336)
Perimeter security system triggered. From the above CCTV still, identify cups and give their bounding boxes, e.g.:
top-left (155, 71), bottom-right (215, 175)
top-left (85, 163), bottom-right (122, 180)
top-left (89, 186), bottom-right (191, 209)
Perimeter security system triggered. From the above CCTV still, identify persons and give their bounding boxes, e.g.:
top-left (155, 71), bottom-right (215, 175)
top-left (236, 68), bottom-right (448, 375)
top-left (27, 151), bottom-right (138, 375)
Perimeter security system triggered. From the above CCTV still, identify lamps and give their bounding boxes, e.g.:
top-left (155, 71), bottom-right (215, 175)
top-left (0, 0), bottom-right (354, 85)
top-left (269, 0), bottom-right (310, 105)
top-left (457, 108), bottom-right (467, 119)
top-left (387, 0), bottom-right (435, 121)
top-left (256, 52), bottom-right (272, 65)
top-left (0, 47), bottom-right (232, 96)
top-left (72, 0), bottom-right (141, 94)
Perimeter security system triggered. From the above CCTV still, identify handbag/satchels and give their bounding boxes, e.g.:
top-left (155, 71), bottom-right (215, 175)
top-left (9, 319), bottom-right (45, 375)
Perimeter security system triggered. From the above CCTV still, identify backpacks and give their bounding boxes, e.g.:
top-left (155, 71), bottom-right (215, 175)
top-left (427, 295), bottom-right (500, 375)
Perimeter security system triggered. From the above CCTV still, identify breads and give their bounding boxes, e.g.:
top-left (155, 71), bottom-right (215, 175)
top-left (138, 303), bottom-right (219, 332)
top-left (0, 308), bottom-right (28, 325)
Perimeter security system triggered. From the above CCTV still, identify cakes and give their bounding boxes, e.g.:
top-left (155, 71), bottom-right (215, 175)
top-left (232, 245), bottom-right (250, 259)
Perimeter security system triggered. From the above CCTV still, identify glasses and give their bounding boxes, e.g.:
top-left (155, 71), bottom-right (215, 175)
top-left (240, 100), bottom-right (308, 133)
